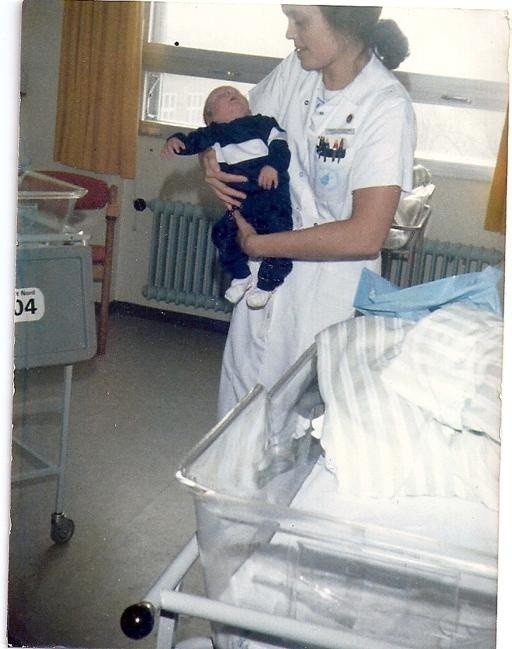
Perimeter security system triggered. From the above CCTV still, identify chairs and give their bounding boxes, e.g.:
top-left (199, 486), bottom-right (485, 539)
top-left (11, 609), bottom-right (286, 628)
top-left (23, 166), bottom-right (120, 358)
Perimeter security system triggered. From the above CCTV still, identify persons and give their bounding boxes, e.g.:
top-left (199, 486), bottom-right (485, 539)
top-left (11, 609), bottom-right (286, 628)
top-left (156, 84), bottom-right (296, 310)
top-left (198, 5), bottom-right (419, 430)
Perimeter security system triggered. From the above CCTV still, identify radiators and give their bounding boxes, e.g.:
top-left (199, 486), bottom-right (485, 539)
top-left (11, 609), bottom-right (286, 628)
top-left (141, 194), bottom-right (235, 314)
top-left (376, 235), bottom-right (506, 288)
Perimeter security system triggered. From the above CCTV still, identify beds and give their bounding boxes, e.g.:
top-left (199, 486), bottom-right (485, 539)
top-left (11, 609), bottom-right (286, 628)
top-left (122, 259), bottom-right (505, 649)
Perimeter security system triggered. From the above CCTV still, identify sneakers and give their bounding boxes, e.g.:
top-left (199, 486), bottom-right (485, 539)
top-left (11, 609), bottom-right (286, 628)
top-left (224, 275), bottom-right (253, 304)
top-left (246, 285), bottom-right (275, 310)
